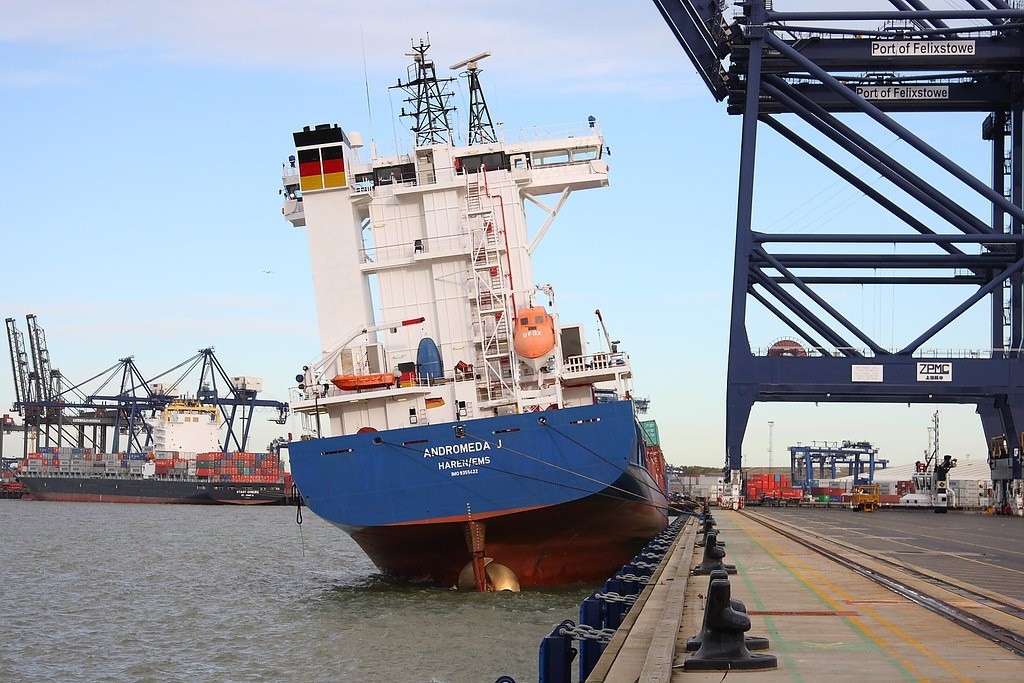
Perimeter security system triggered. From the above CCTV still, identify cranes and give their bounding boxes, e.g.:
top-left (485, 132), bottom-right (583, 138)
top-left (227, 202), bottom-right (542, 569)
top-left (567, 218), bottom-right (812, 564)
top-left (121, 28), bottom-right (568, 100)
top-left (4, 313), bottom-right (293, 448)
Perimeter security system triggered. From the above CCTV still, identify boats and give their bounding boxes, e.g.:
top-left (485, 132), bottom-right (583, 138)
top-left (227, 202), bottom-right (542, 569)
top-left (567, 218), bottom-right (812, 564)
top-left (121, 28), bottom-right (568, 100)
top-left (330, 371), bottom-right (396, 391)
top-left (3, 445), bottom-right (292, 506)
top-left (278, 28), bottom-right (670, 593)
